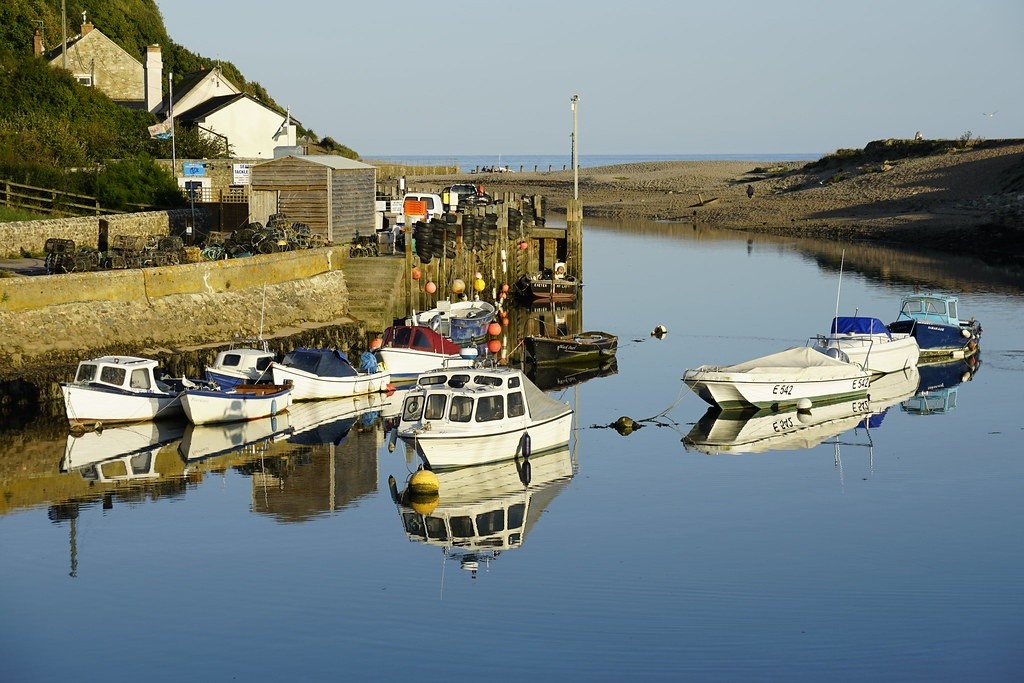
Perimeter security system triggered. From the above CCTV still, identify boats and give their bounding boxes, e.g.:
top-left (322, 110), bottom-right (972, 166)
top-left (679, 363), bottom-right (922, 489)
top-left (270, 344), bottom-right (392, 403)
top-left (525, 267), bottom-right (580, 299)
top-left (58, 355), bottom-right (185, 427)
top-left (395, 308), bottom-right (575, 471)
top-left (522, 317), bottom-right (620, 367)
top-left (885, 289), bottom-right (983, 358)
top-left (58, 416), bottom-right (187, 484)
top-left (176, 376), bottom-right (294, 425)
top-left (204, 279), bottom-right (283, 391)
top-left (522, 352), bottom-right (618, 394)
top-left (681, 249), bottom-right (922, 412)
top-left (523, 296), bottom-right (580, 322)
top-left (267, 391), bottom-right (394, 446)
top-left (389, 446), bottom-right (574, 602)
top-left (370, 283), bottom-right (499, 384)
top-left (178, 412), bottom-right (294, 483)
top-left (900, 341), bottom-right (983, 416)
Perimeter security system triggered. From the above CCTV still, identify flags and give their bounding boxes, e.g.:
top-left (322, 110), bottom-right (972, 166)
top-left (148, 116), bottom-right (172, 139)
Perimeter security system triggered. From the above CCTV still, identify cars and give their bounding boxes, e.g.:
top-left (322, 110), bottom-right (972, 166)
top-left (395, 183), bottom-right (502, 233)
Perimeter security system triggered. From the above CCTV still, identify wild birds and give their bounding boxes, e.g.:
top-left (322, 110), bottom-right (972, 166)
top-left (983, 110), bottom-right (999, 118)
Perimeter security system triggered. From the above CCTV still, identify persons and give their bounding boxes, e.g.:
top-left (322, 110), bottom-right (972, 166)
top-left (746, 185), bottom-right (754, 198)
